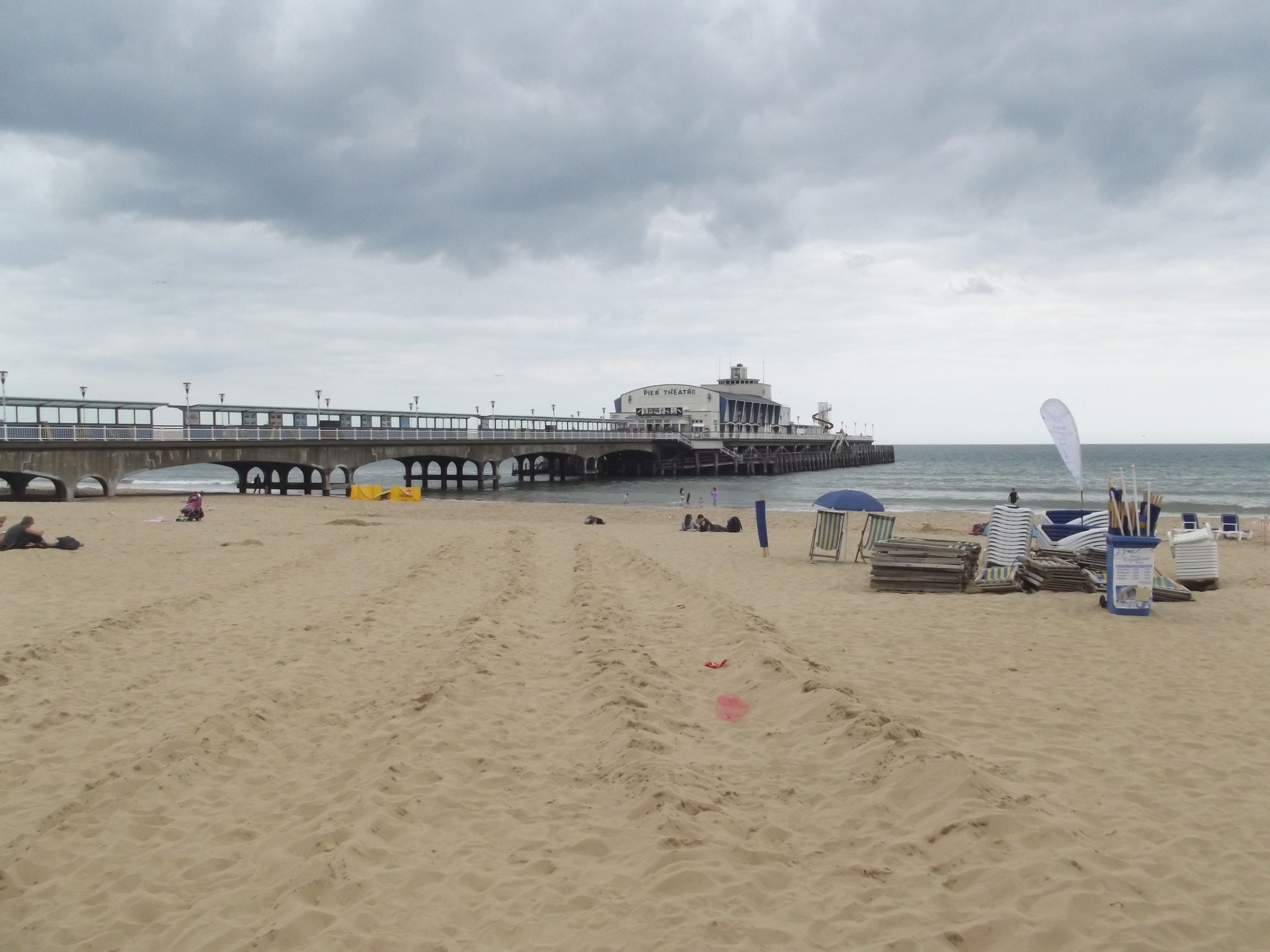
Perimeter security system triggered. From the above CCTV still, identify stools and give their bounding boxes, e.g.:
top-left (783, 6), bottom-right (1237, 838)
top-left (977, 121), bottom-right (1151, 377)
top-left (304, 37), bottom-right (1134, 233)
top-left (868, 536), bottom-right (1108, 595)
top-left (1167, 522), bottom-right (1219, 590)
top-left (1214, 513), bottom-right (1252, 541)
top-left (983, 503), bottom-right (1110, 570)
top-left (1178, 512), bottom-right (1200, 530)
top-left (1153, 576), bottom-right (1191, 603)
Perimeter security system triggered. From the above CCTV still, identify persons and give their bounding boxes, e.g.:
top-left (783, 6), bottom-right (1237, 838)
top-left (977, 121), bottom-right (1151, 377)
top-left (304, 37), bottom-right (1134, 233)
top-left (0, 515), bottom-right (55, 551)
top-left (682, 514), bottom-right (700, 531)
top-left (253, 474), bottom-right (262, 494)
top-left (711, 487), bottom-right (718, 506)
top-left (1009, 487), bottom-right (1020, 504)
top-left (678, 487), bottom-right (688, 503)
top-left (698, 497), bottom-right (705, 509)
top-left (696, 513), bottom-right (729, 532)
top-left (623, 493), bottom-right (628, 506)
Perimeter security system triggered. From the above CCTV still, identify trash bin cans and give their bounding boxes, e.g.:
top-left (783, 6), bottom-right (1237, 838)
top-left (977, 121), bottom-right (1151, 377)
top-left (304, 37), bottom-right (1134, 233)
top-left (1100, 531), bottom-right (1162, 617)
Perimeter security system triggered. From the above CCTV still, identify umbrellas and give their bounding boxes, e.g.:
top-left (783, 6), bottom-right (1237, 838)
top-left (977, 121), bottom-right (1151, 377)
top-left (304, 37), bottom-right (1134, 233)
top-left (1105, 476), bottom-right (1164, 538)
top-left (812, 489), bottom-right (886, 563)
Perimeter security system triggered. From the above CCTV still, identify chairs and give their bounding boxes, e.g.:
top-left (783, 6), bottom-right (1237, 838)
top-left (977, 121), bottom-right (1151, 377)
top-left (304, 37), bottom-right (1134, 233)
top-left (855, 513), bottom-right (896, 564)
top-left (808, 509), bottom-right (845, 563)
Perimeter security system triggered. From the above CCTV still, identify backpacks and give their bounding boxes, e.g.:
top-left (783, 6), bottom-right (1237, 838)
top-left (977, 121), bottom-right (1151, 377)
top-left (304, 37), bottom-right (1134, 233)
top-left (727, 516), bottom-right (743, 532)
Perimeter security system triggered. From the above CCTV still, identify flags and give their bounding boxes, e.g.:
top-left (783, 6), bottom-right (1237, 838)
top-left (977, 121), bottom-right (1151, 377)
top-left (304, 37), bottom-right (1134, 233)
top-left (1039, 398), bottom-right (1085, 493)
